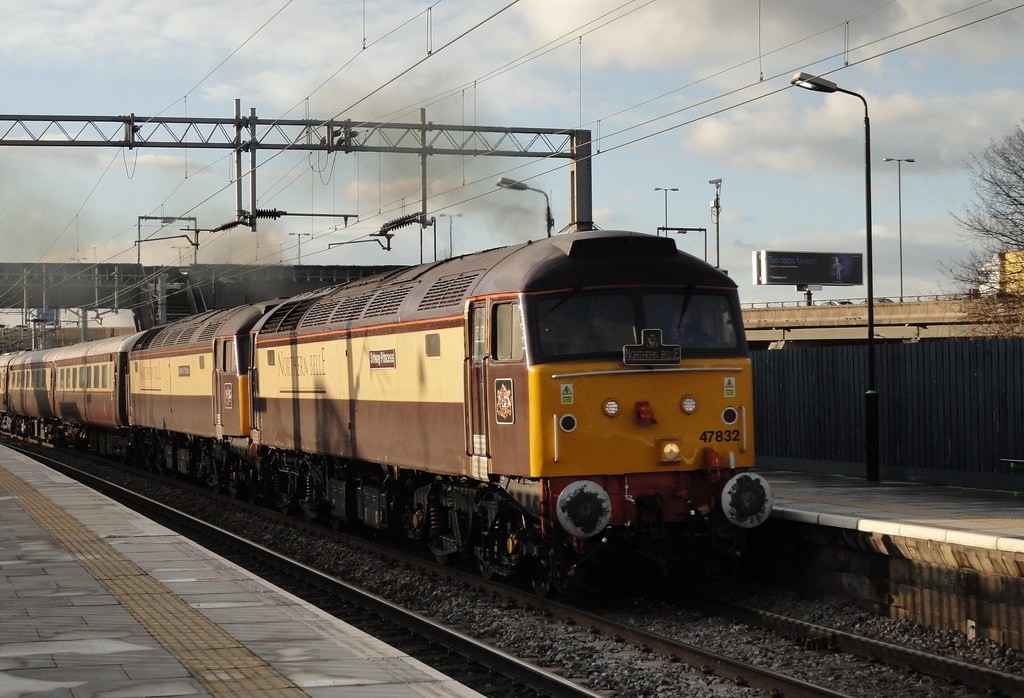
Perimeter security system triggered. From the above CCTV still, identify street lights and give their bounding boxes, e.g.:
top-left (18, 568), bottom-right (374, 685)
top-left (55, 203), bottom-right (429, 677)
top-left (439, 212), bottom-right (463, 261)
top-left (178, 268), bottom-right (215, 311)
top-left (172, 246), bottom-right (191, 267)
top-left (884, 157), bottom-right (916, 304)
top-left (790, 70), bottom-right (882, 487)
top-left (288, 232), bottom-right (310, 265)
top-left (494, 177), bottom-right (556, 240)
top-left (651, 186), bottom-right (680, 237)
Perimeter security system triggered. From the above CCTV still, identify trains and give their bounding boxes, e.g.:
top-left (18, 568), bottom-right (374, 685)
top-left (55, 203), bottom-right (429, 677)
top-left (0, 226), bottom-right (773, 587)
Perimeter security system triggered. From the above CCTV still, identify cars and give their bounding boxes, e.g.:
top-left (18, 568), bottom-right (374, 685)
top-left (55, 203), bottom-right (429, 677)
top-left (859, 299), bottom-right (895, 303)
top-left (818, 301), bottom-right (855, 305)
